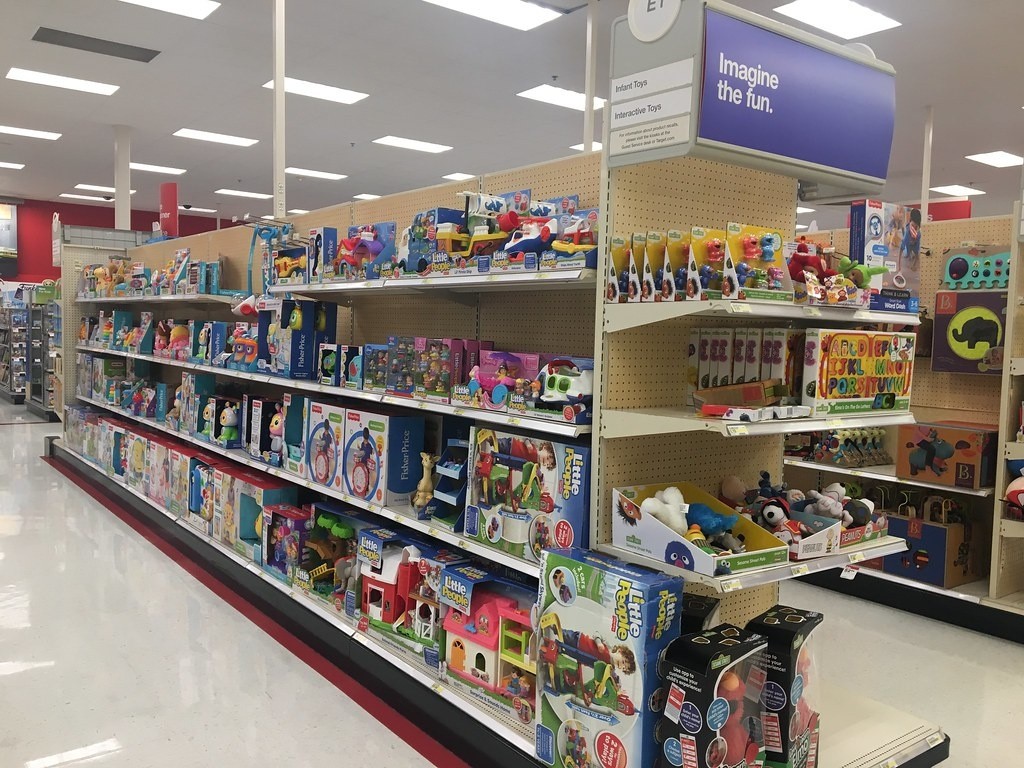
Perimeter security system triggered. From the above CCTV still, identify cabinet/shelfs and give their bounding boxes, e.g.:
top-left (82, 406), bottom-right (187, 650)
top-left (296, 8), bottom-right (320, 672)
top-left (980, 358), bottom-right (1024, 616)
top-left (0, 307), bottom-right (26, 403)
top-left (782, 447), bottom-right (996, 605)
top-left (589, 155), bottom-right (951, 768)
top-left (45, 150), bottom-right (602, 768)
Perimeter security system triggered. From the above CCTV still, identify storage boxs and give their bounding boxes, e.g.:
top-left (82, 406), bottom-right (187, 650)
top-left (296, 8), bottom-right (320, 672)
top-left (686, 328), bottom-right (917, 418)
top-left (534, 547), bottom-right (685, 768)
top-left (282, 393), bottom-right (590, 566)
top-left (660, 592), bottom-right (824, 768)
top-left (854, 504), bottom-right (989, 590)
top-left (896, 420), bottom-right (999, 490)
top-left (306, 227), bottom-right (337, 285)
top-left (256, 299), bottom-right (591, 406)
top-left (62, 405), bottom-right (539, 730)
top-left (272, 246), bottom-right (310, 285)
top-left (850, 198), bottom-right (921, 299)
top-left (931, 290), bottom-right (1007, 376)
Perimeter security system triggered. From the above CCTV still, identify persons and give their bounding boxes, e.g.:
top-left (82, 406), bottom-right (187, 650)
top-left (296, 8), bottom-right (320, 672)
top-left (367, 342), bottom-right (456, 396)
top-left (354, 428), bottom-right (374, 492)
top-left (487, 436), bottom-right (556, 483)
top-left (544, 624), bottom-right (636, 686)
top-left (319, 419), bottom-right (333, 478)
top-left (507, 666), bottom-right (531, 697)
top-left (495, 365), bottom-right (511, 381)
top-left (883, 204), bottom-right (906, 248)
top-left (897, 207), bottom-right (922, 274)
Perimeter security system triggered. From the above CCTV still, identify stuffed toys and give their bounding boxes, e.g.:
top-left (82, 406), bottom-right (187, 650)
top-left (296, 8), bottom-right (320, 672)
top-left (639, 470), bottom-right (883, 557)
top-left (792, 237), bottom-right (894, 288)
